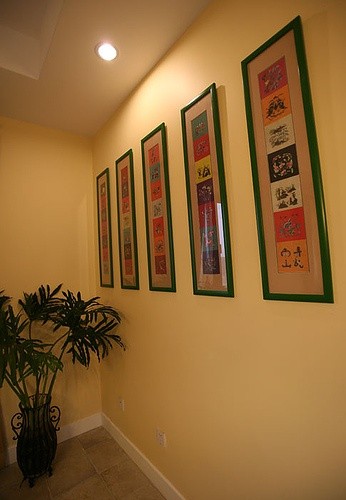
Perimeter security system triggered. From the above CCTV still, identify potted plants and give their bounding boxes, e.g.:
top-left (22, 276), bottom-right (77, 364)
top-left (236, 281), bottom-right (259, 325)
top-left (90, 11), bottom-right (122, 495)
top-left (0, 283), bottom-right (127, 490)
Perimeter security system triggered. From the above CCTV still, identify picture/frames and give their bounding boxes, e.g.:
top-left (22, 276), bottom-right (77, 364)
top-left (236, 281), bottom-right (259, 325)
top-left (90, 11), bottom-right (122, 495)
top-left (241, 15), bottom-right (335, 304)
top-left (96, 168), bottom-right (114, 288)
top-left (180, 82), bottom-right (235, 298)
top-left (116, 149), bottom-right (140, 290)
top-left (140, 121), bottom-right (177, 293)
